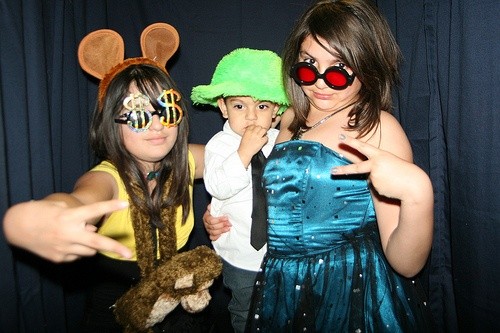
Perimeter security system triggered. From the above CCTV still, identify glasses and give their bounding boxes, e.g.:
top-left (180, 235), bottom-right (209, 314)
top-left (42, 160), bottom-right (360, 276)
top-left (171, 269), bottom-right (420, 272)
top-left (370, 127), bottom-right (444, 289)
top-left (116, 106), bottom-right (183, 132)
top-left (290, 60), bottom-right (356, 90)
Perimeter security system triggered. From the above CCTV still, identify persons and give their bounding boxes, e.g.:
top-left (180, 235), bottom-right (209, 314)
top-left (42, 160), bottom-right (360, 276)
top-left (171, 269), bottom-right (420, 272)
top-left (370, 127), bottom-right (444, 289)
top-left (202, 0), bottom-right (435, 333)
top-left (190, 48), bottom-right (290, 333)
top-left (3, 22), bottom-right (282, 333)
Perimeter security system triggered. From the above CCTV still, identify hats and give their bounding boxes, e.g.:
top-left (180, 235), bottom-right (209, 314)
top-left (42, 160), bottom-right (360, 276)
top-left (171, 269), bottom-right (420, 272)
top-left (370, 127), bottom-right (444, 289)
top-left (191, 48), bottom-right (290, 115)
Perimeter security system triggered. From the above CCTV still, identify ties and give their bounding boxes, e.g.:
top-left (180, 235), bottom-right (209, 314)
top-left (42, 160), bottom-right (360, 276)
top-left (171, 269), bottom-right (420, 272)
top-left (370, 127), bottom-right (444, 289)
top-left (251, 150), bottom-right (267, 251)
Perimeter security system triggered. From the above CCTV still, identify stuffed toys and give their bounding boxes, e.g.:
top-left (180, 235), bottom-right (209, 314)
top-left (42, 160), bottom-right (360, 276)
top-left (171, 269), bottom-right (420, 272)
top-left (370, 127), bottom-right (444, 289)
top-left (111, 156), bottom-right (223, 333)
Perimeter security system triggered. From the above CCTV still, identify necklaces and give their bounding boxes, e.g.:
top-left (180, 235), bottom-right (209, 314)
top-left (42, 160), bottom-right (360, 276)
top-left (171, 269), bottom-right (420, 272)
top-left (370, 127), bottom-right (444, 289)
top-left (300, 98), bottom-right (357, 133)
top-left (146, 168), bottom-right (159, 181)
